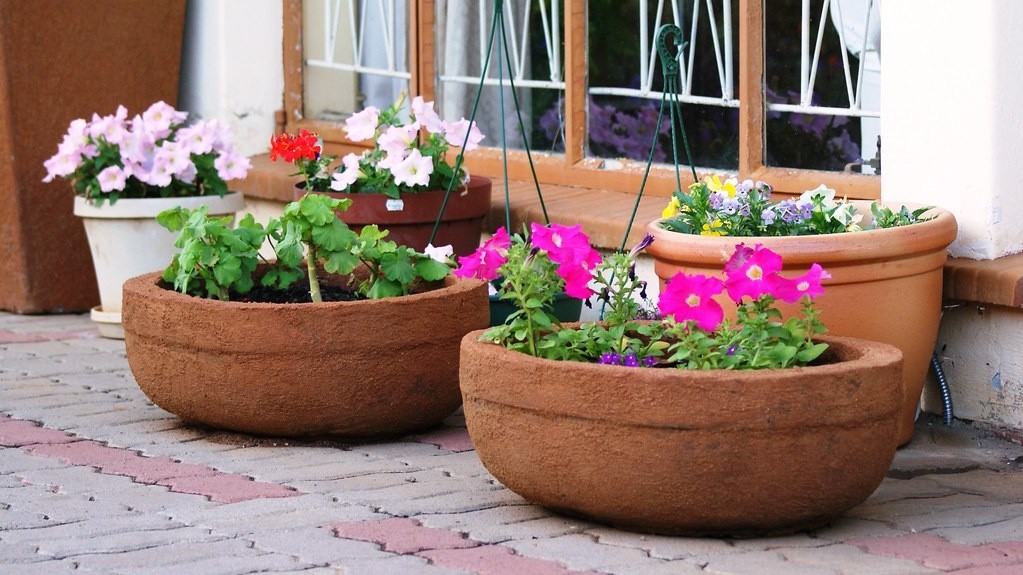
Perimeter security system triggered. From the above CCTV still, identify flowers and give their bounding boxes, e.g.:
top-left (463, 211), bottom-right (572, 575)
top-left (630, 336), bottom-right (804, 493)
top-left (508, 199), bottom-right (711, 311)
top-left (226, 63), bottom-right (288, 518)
top-left (41, 96), bottom-right (937, 375)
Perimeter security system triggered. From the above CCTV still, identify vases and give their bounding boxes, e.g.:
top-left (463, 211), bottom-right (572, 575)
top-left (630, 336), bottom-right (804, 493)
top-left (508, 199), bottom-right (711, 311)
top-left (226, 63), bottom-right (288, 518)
top-left (123, 255), bottom-right (491, 439)
top-left (489, 291), bottom-right (582, 324)
top-left (459, 319), bottom-right (905, 532)
top-left (72, 189), bottom-right (244, 312)
top-left (647, 203), bottom-right (955, 445)
top-left (294, 173), bottom-right (493, 257)
